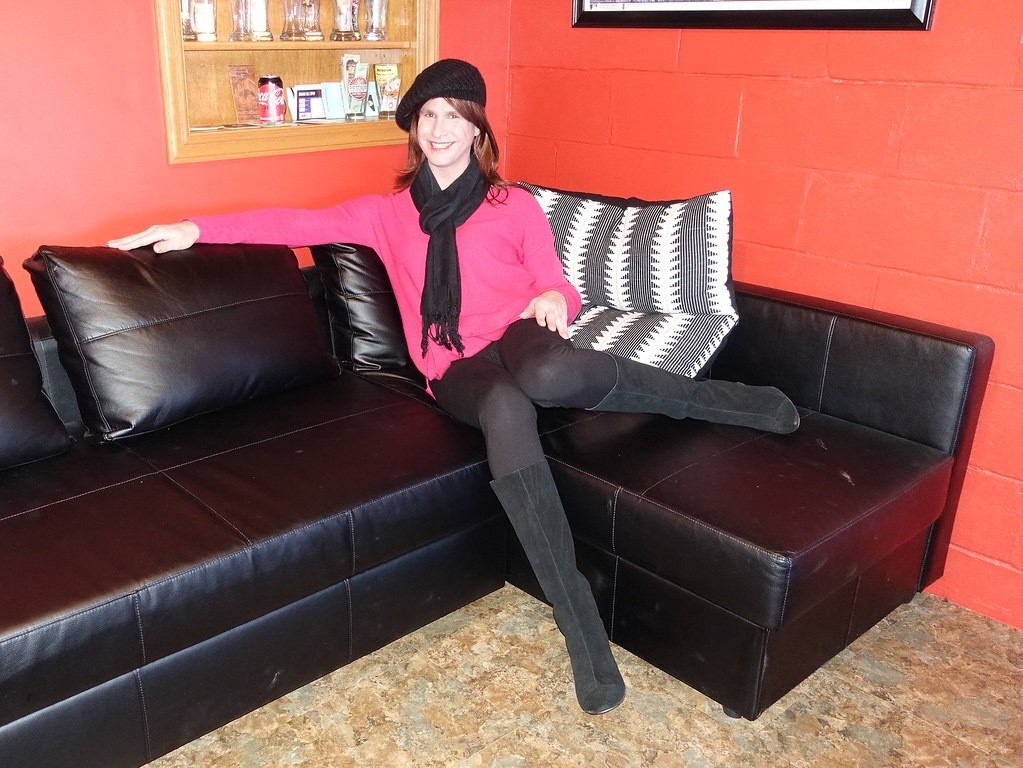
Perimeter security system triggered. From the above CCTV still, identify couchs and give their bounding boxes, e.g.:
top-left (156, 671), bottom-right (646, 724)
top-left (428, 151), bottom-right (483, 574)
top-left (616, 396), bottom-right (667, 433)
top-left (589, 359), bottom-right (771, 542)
top-left (0, 225), bottom-right (997, 768)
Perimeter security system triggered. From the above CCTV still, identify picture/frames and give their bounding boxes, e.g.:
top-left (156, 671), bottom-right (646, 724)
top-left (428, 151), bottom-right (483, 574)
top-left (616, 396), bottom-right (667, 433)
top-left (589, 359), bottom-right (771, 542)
top-left (571, 1), bottom-right (936, 31)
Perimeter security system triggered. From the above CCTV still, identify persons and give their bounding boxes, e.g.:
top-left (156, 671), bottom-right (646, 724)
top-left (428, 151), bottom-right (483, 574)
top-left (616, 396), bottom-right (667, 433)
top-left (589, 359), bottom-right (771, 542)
top-left (107, 59), bottom-right (801, 714)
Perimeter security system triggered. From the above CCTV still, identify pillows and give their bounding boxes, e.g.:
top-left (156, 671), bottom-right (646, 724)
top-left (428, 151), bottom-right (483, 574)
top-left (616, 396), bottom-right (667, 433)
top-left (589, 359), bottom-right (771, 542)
top-left (0, 182), bottom-right (740, 467)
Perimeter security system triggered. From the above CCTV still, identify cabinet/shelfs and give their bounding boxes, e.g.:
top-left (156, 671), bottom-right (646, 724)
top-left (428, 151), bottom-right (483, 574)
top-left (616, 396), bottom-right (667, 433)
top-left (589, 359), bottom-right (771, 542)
top-left (155, 0), bottom-right (441, 165)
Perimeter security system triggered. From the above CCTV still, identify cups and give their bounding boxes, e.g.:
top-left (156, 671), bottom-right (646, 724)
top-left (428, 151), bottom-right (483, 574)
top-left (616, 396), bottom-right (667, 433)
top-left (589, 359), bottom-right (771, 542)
top-left (229, 0), bottom-right (274, 42)
top-left (374, 62), bottom-right (404, 118)
top-left (338, 62), bottom-right (371, 121)
top-left (329, 0), bottom-right (362, 41)
top-left (278, 0), bottom-right (325, 42)
top-left (180, 0), bottom-right (218, 41)
top-left (363, 0), bottom-right (391, 42)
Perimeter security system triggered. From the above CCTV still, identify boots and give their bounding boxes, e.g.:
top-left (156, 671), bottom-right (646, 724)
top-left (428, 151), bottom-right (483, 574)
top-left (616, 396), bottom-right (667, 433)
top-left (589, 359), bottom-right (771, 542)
top-left (490, 458), bottom-right (626, 716)
top-left (585, 352), bottom-right (799, 434)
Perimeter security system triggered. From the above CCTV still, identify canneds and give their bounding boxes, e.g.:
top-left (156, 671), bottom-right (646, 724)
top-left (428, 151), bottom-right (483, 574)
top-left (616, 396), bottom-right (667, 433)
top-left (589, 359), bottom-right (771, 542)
top-left (258, 75), bottom-right (284, 125)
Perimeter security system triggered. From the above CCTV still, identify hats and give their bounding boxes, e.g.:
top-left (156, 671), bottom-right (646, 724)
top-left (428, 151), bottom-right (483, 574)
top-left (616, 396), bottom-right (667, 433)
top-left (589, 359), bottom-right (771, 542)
top-left (395, 59), bottom-right (486, 132)
